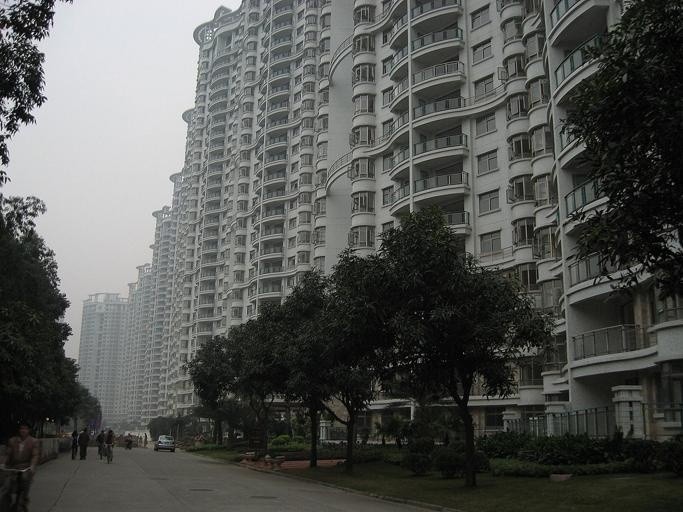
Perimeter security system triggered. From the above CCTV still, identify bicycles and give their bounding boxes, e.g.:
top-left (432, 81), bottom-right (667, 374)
top-left (0, 466), bottom-right (33, 512)
top-left (96, 443), bottom-right (104, 460)
top-left (104, 442), bottom-right (114, 463)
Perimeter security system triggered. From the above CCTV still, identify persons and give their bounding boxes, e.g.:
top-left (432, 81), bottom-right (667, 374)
top-left (72, 428), bottom-right (148, 464)
top-left (0, 420), bottom-right (41, 512)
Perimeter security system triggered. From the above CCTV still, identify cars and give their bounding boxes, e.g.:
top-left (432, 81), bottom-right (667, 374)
top-left (154, 434), bottom-right (175, 452)
